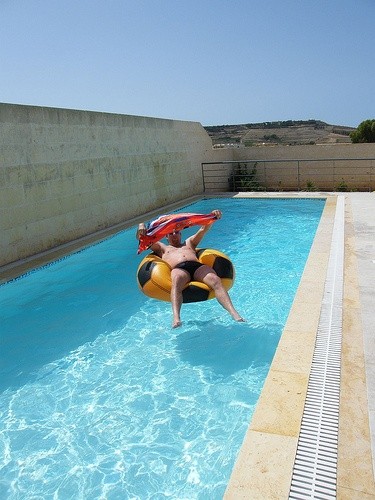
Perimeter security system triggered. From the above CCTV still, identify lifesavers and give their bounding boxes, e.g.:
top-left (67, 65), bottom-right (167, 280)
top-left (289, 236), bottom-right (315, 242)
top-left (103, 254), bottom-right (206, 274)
top-left (135, 246), bottom-right (235, 303)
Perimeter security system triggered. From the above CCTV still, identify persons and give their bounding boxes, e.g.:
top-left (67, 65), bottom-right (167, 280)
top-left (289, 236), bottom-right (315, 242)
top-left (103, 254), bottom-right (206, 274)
top-left (136, 209), bottom-right (247, 329)
